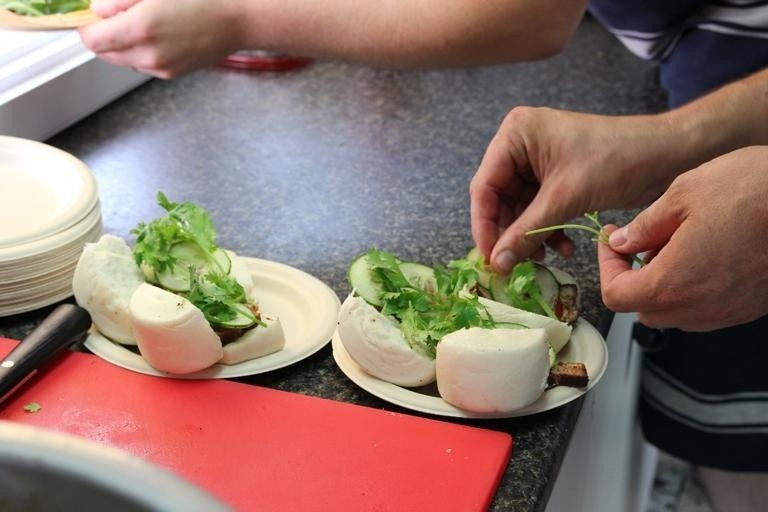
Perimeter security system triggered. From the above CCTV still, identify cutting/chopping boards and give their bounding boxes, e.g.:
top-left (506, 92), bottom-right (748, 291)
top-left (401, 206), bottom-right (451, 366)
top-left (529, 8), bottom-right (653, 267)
top-left (1, 333), bottom-right (515, 512)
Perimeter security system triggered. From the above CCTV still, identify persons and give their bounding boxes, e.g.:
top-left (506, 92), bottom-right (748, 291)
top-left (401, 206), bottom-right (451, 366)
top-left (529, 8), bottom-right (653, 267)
top-left (469, 69), bottom-right (768, 334)
top-left (78, 0), bottom-right (768, 474)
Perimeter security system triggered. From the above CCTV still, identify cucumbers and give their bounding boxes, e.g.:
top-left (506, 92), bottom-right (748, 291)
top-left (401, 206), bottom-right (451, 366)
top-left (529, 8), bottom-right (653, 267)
top-left (347, 252), bottom-right (438, 307)
top-left (468, 246), bottom-right (560, 317)
top-left (154, 239), bottom-right (232, 292)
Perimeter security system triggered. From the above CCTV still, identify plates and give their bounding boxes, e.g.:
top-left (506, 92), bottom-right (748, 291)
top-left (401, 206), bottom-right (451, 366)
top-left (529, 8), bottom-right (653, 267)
top-left (332, 273), bottom-right (609, 422)
top-left (0, 128), bottom-right (105, 313)
top-left (80, 253), bottom-right (346, 383)
